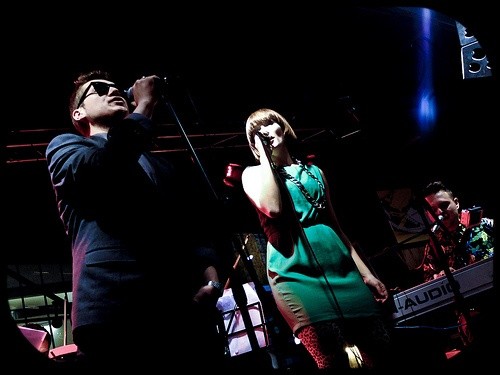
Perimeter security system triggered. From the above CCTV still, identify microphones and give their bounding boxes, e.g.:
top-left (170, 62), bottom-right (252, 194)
top-left (256, 132), bottom-right (270, 146)
top-left (432, 215), bottom-right (444, 232)
top-left (127, 77), bottom-right (168, 102)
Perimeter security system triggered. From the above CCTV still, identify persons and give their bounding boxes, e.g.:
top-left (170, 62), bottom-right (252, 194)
top-left (46, 73), bottom-right (231, 375)
top-left (242, 109), bottom-right (389, 374)
top-left (418, 181), bottom-right (496, 349)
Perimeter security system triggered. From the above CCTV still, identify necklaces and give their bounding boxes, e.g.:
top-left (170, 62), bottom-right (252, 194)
top-left (279, 162), bottom-right (330, 211)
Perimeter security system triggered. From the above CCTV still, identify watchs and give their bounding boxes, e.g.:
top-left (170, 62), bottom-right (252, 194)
top-left (208, 278), bottom-right (226, 297)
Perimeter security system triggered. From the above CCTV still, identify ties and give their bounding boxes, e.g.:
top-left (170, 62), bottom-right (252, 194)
top-left (137, 154), bottom-right (158, 186)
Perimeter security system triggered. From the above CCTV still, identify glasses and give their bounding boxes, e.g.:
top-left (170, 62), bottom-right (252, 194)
top-left (77, 81), bottom-right (127, 110)
